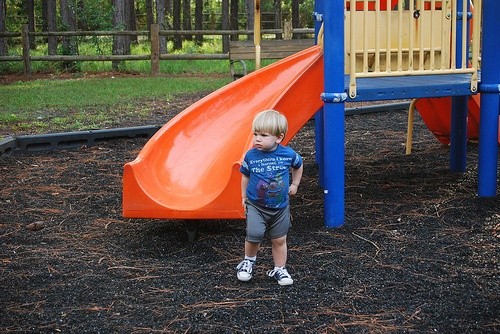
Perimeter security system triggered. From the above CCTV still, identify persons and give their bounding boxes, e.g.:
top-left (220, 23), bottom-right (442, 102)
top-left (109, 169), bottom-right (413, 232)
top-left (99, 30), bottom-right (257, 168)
top-left (236, 109), bottom-right (305, 286)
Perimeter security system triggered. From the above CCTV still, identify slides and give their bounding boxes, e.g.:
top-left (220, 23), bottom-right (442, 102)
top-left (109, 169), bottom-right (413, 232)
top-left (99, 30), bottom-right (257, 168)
top-left (122, 45), bottom-right (324, 220)
top-left (413, 60), bottom-right (500, 145)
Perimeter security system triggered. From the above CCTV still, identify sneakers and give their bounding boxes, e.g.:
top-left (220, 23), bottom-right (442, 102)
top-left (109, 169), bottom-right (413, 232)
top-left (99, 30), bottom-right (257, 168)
top-left (271, 265), bottom-right (294, 287)
top-left (238, 260), bottom-right (254, 282)
